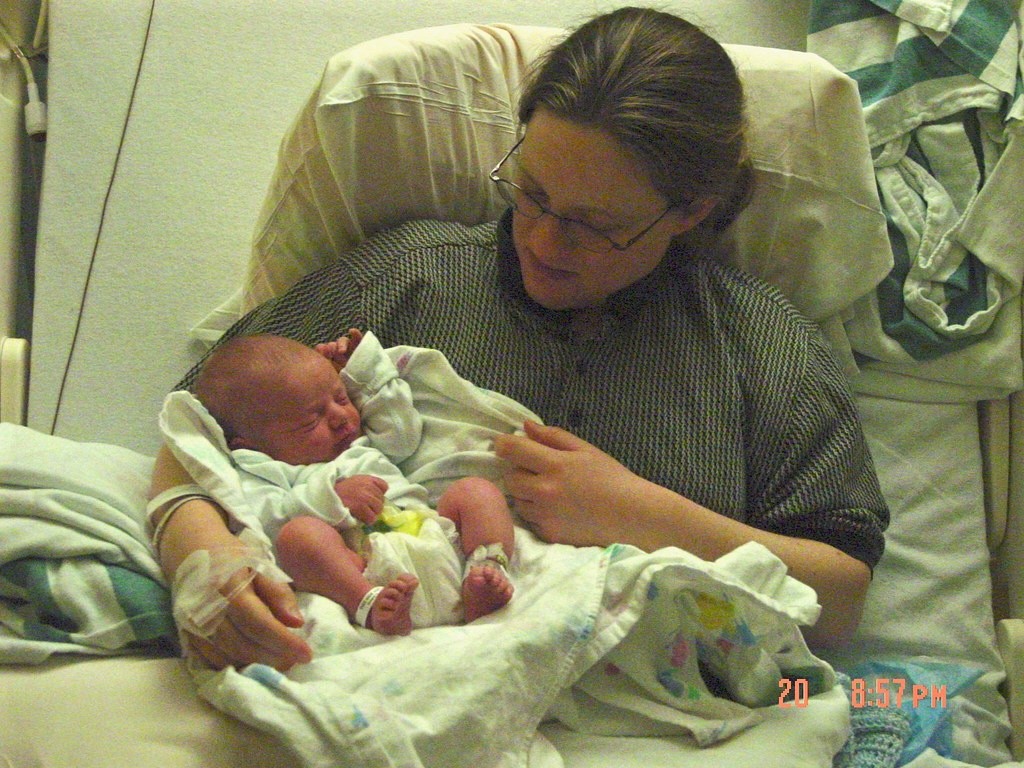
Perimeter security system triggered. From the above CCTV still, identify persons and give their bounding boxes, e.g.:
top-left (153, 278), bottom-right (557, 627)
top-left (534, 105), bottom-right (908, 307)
top-left (143, 3), bottom-right (889, 768)
top-left (188, 325), bottom-right (514, 637)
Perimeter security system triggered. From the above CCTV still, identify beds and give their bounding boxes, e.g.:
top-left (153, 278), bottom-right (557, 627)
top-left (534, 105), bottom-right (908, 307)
top-left (0, 0), bottom-right (1024, 768)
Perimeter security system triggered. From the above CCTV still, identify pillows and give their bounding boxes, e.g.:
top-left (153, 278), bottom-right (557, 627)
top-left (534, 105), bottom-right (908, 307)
top-left (182, 22), bottom-right (894, 398)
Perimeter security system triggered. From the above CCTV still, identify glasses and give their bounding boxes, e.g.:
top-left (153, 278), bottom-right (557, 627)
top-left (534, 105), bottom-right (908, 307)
top-left (489, 136), bottom-right (675, 254)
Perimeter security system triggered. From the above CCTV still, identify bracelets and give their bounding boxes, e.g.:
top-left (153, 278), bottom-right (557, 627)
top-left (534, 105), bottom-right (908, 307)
top-left (144, 481), bottom-right (230, 555)
